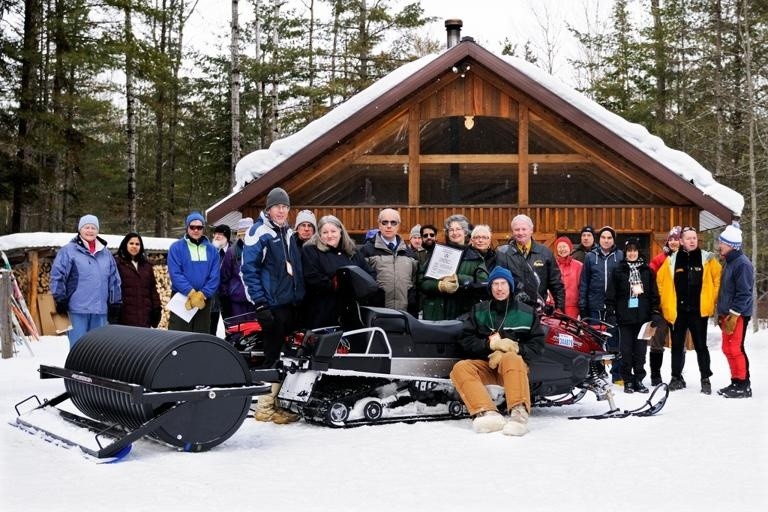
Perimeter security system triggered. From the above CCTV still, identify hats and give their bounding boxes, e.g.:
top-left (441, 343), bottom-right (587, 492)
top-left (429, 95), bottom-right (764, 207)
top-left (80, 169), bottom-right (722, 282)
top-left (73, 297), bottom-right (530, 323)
top-left (237, 217), bottom-right (255, 237)
top-left (624, 235), bottom-right (642, 247)
top-left (214, 223), bottom-right (233, 240)
top-left (184, 210), bottom-right (207, 227)
top-left (580, 224), bottom-right (597, 238)
top-left (598, 224), bottom-right (617, 238)
top-left (718, 220), bottom-right (743, 247)
top-left (553, 233), bottom-right (574, 256)
top-left (294, 208), bottom-right (318, 232)
top-left (76, 213), bottom-right (100, 230)
top-left (365, 228), bottom-right (381, 239)
top-left (486, 265), bottom-right (516, 292)
top-left (265, 187), bottom-right (291, 212)
top-left (409, 222), bottom-right (423, 239)
top-left (665, 224), bottom-right (682, 241)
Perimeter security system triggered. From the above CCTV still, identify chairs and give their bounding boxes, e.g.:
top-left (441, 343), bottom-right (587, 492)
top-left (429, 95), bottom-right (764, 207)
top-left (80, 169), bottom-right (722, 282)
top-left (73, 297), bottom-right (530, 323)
top-left (334, 262), bottom-right (405, 335)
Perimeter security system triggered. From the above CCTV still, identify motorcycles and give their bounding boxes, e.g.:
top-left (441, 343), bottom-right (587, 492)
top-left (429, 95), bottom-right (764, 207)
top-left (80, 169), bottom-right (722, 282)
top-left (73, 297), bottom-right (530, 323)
top-left (274, 294), bottom-right (672, 428)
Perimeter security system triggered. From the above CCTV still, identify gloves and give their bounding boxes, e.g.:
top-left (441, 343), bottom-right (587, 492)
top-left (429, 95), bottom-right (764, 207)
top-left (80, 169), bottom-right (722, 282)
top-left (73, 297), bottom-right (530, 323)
top-left (107, 303), bottom-right (120, 324)
top-left (151, 307), bottom-right (161, 328)
top-left (649, 310), bottom-right (662, 328)
top-left (56, 299), bottom-right (70, 319)
top-left (489, 337), bottom-right (520, 354)
top-left (438, 274), bottom-right (460, 294)
top-left (255, 305), bottom-right (279, 338)
top-left (487, 350), bottom-right (505, 369)
top-left (185, 288), bottom-right (208, 311)
top-left (219, 295), bottom-right (232, 320)
top-left (724, 309), bottom-right (741, 336)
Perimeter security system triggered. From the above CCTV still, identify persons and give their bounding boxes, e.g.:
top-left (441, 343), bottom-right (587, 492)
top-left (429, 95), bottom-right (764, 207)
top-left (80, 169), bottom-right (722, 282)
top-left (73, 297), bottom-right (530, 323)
top-left (654, 228), bottom-right (721, 397)
top-left (578, 225), bottom-right (626, 385)
top-left (604, 237), bottom-right (663, 394)
top-left (167, 186), bottom-right (599, 435)
top-left (49, 215), bottom-right (123, 350)
top-left (112, 233), bottom-right (163, 328)
top-left (649, 225), bottom-right (682, 386)
top-left (712, 223), bottom-right (755, 400)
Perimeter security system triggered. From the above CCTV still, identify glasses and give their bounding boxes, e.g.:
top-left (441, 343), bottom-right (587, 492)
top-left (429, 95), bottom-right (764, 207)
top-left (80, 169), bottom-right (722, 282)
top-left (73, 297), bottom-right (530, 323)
top-left (680, 225), bottom-right (698, 233)
top-left (189, 225), bottom-right (205, 231)
top-left (379, 220), bottom-right (401, 226)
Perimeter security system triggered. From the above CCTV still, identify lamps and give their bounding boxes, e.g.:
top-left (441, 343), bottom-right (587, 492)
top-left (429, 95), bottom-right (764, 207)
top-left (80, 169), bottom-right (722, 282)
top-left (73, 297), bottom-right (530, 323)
top-left (452, 63), bottom-right (472, 79)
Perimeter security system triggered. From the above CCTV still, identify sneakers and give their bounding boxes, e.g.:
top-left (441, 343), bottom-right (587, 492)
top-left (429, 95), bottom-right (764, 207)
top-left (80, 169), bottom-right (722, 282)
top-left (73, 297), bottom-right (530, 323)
top-left (623, 381), bottom-right (635, 394)
top-left (717, 377), bottom-right (753, 398)
top-left (503, 409), bottom-right (530, 436)
top-left (651, 374), bottom-right (663, 387)
top-left (473, 411), bottom-right (505, 433)
top-left (663, 375), bottom-right (687, 391)
top-left (635, 379), bottom-right (649, 393)
top-left (612, 379), bottom-right (625, 386)
top-left (701, 378), bottom-right (712, 395)
